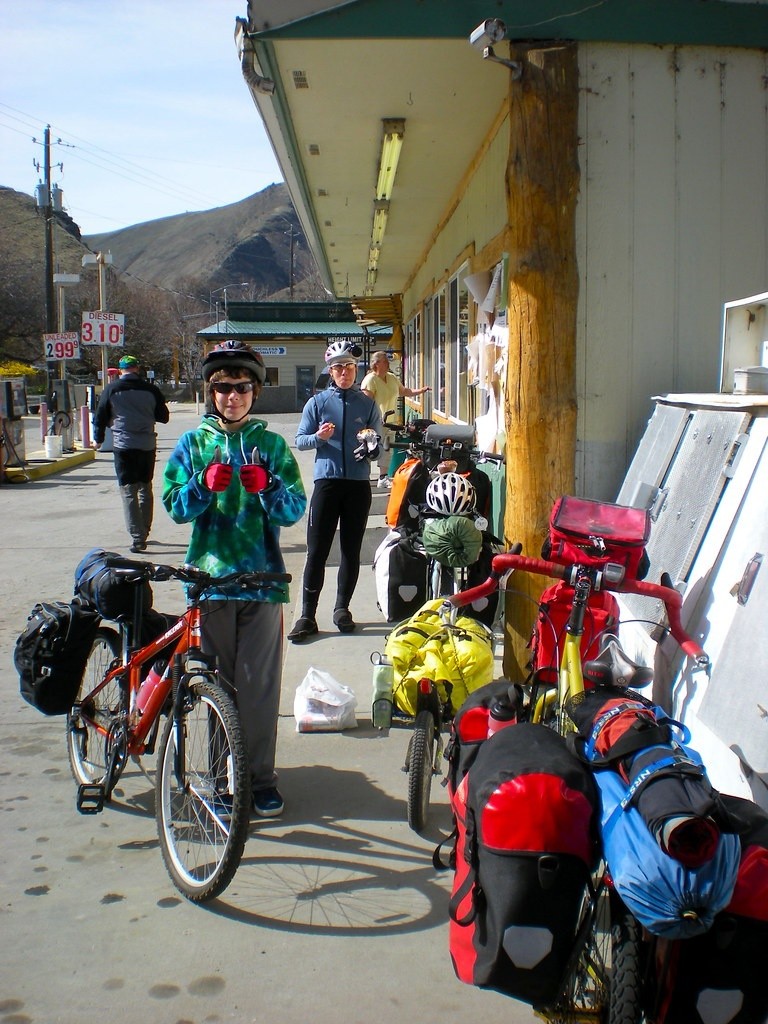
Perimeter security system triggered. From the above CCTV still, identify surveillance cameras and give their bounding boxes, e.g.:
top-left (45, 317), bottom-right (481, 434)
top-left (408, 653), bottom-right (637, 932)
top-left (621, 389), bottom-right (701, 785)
top-left (469, 18), bottom-right (508, 48)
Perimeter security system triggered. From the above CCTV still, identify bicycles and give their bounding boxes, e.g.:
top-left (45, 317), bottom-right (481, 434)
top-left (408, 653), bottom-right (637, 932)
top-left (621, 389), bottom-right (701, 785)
top-left (65, 559), bottom-right (292, 905)
top-left (378, 409), bottom-right (508, 834)
top-left (440, 494), bottom-right (713, 1024)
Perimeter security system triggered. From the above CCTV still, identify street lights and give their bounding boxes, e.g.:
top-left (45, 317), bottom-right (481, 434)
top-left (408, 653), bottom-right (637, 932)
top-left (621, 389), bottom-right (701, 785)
top-left (82, 251), bottom-right (114, 392)
top-left (209, 283), bottom-right (249, 317)
top-left (53, 273), bottom-right (81, 381)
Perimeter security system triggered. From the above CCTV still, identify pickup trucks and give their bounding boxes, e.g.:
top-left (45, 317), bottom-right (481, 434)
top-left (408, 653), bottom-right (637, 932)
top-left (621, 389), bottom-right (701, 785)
top-left (26, 394), bottom-right (48, 415)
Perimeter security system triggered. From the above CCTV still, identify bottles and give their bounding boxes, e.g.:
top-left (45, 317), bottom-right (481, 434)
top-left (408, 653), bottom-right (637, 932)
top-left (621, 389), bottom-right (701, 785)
top-left (136, 658), bottom-right (167, 709)
top-left (372, 654), bottom-right (391, 729)
top-left (487, 700), bottom-right (517, 741)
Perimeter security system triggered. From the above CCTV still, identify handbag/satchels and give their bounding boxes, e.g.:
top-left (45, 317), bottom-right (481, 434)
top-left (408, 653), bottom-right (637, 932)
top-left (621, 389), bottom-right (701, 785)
top-left (383, 599), bottom-right (494, 720)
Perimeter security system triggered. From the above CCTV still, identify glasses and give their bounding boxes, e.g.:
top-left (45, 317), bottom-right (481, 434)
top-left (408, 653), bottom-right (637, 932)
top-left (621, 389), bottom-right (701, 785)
top-left (329, 362), bottom-right (358, 369)
top-left (211, 381), bottom-right (255, 394)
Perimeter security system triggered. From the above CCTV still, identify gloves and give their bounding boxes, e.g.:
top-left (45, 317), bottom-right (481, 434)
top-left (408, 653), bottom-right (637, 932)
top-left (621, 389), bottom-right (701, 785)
top-left (201, 445), bottom-right (233, 492)
top-left (353, 435), bottom-right (381, 463)
top-left (240, 446), bottom-right (273, 493)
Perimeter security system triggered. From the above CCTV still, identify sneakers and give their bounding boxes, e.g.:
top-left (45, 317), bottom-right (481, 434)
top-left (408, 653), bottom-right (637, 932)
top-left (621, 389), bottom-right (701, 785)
top-left (251, 785), bottom-right (285, 818)
top-left (213, 783), bottom-right (233, 821)
top-left (376, 475), bottom-right (394, 488)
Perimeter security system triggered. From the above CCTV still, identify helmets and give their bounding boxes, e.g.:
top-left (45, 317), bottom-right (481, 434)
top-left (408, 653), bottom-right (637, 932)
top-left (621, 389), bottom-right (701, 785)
top-left (324, 341), bottom-right (360, 365)
top-left (426, 472), bottom-right (477, 515)
top-left (202, 339), bottom-right (266, 385)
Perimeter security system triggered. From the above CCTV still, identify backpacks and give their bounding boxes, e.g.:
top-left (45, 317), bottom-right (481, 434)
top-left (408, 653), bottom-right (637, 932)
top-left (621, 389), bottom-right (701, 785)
top-left (431, 723), bottom-right (600, 1010)
top-left (459, 468), bottom-right (505, 627)
top-left (531, 580), bottom-right (620, 689)
top-left (372, 525), bottom-right (433, 623)
top-left (384, 458), bottom-right (429, 530)
top-left (638, 789), bottom-right (767, 1024)
top-left (14, 601), bottom-right (100, 716)
top-left (445, 674), bottom-right (525, 871)
top-left (126, 608), bottom-right (200, 718)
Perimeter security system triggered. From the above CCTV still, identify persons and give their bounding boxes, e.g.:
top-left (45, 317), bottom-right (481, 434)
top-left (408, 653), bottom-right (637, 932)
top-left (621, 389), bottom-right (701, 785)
top-left (92, 355), bottom-right (170, 553)
top-left (360, 351), bottom-right (432, 489)
top-left (162, 338), bottom-right (307, 822)
top-left (286, 340), bottom-right (384, 641)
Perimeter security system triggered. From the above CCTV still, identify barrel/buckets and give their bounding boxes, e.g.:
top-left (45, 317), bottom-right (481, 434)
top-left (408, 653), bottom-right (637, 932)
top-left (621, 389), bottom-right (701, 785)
top-left (44, 436), bottom-right (63, 458)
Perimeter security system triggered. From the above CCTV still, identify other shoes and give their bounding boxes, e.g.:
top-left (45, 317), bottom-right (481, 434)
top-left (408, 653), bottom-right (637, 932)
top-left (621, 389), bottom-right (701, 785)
top-left (129, 542), bottom-right (147, 551)
top-left (333, 607), bottom-right (357, 633)
top-left (287, 615), bottom-right (319, 640)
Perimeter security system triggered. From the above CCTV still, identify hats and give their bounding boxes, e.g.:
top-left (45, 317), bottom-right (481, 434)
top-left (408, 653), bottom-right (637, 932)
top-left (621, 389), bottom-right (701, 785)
top-left (119, 355), bottom-right (141, 370)
